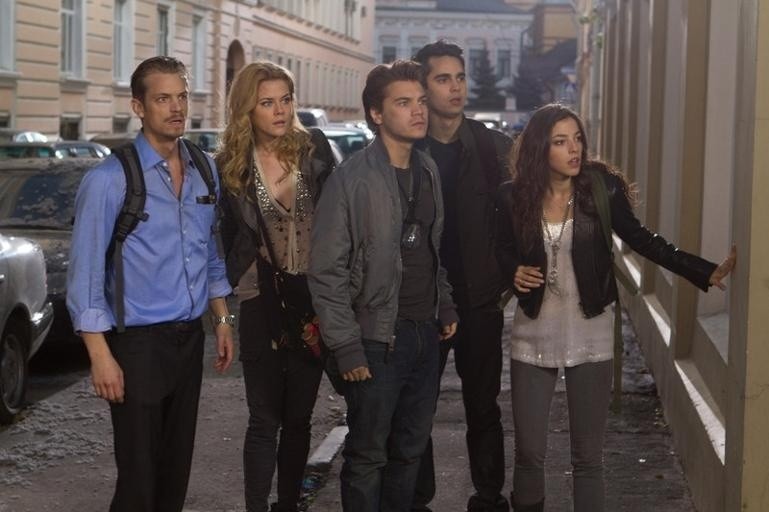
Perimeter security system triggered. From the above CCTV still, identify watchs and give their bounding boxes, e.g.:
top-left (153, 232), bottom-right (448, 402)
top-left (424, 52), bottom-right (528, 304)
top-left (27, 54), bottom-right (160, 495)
top-left (215, 315), bottom-right (236, 328)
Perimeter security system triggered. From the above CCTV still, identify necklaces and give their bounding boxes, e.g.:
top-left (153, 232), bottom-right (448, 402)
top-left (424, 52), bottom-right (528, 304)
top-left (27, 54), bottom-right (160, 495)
top-left (540, 193), bottom-right (575, 295)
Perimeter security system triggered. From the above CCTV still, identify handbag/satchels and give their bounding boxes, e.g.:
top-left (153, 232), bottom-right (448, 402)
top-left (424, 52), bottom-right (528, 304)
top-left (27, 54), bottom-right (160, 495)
top-left (253, 260), bottom-right (331, 379)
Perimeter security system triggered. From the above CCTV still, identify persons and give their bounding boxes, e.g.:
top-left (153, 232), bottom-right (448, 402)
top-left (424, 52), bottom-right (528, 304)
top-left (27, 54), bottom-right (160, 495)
top-left (215, 64), bottom-right (335, 512)
top-left (412, 44), bottom-right (516, 512)
top-left (66, 58), bottom-right (236, 512)
top-left (307, 64), bottom-right (461, 512)
top-left (497, 104), bottom-right (737, 512)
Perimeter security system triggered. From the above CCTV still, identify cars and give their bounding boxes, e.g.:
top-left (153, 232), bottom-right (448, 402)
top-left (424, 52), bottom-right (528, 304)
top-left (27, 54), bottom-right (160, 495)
top-left (183, 128), bottom-right (227, 162)
top-left (1, 231), bottom-right (54, 418)
top-left (0, 158), bottom-right (109, 359)
top-left (472, 110), bottom-right (525, 134)
top-left (0, 130), bottom-right (137, 158)
top-left (293, 109), bottom-right (372, 166)
